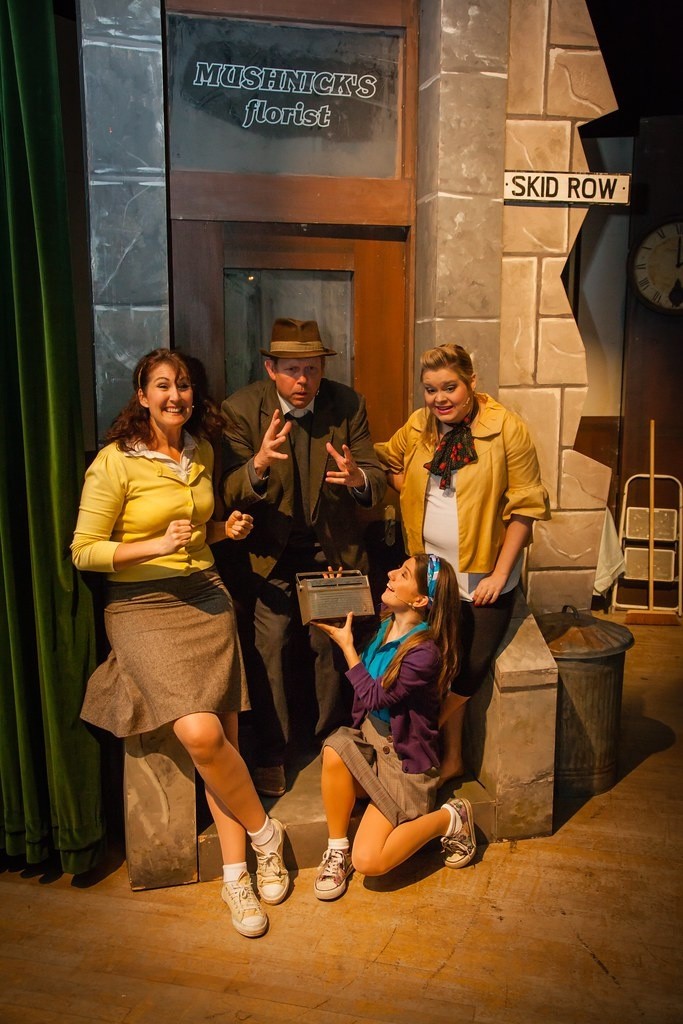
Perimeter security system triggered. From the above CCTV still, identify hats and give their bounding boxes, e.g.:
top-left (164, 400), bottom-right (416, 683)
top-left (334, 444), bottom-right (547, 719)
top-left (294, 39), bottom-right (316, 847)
top-left (259, 317), bottom-right (338, 361)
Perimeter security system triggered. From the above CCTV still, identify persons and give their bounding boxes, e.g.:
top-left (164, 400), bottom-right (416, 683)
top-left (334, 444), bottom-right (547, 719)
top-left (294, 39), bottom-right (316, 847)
top-left (217, 317), bottom-right (388, 799)
top-left (367, 343), bottom-right (552, 793)
top-left (312, 552), bottom-right (480, 901)
top-left (70, 346), bottom-right (294, 937)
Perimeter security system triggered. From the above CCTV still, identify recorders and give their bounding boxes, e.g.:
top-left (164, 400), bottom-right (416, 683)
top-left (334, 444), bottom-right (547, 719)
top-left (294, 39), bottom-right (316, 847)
top-left (296, 570), bottom-right (375, 625)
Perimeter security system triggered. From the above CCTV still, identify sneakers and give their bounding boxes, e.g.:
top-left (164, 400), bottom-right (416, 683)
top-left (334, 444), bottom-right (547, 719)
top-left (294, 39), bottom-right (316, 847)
top-left (314, 849), bottom-right (356, 902)
top-left (444, 798), bottom-right (476, 869)
top-left (221, 819), bottom-right (290, 938)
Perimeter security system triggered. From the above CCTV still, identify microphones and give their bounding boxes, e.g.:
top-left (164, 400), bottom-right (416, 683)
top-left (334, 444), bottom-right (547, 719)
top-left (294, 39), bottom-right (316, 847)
top-left (394, 593), bottom-right (412, 607)
top-left (462, 393), bottom-right (471, 408)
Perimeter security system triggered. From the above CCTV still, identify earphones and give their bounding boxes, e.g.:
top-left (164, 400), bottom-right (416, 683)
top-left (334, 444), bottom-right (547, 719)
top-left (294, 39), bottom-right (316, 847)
top-left (418, 598), bottom-right (427, 605)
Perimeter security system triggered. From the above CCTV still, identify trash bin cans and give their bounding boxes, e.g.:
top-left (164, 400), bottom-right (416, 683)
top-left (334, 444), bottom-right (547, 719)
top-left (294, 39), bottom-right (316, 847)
top-left (537, 603), bottom-right (636, 795)
